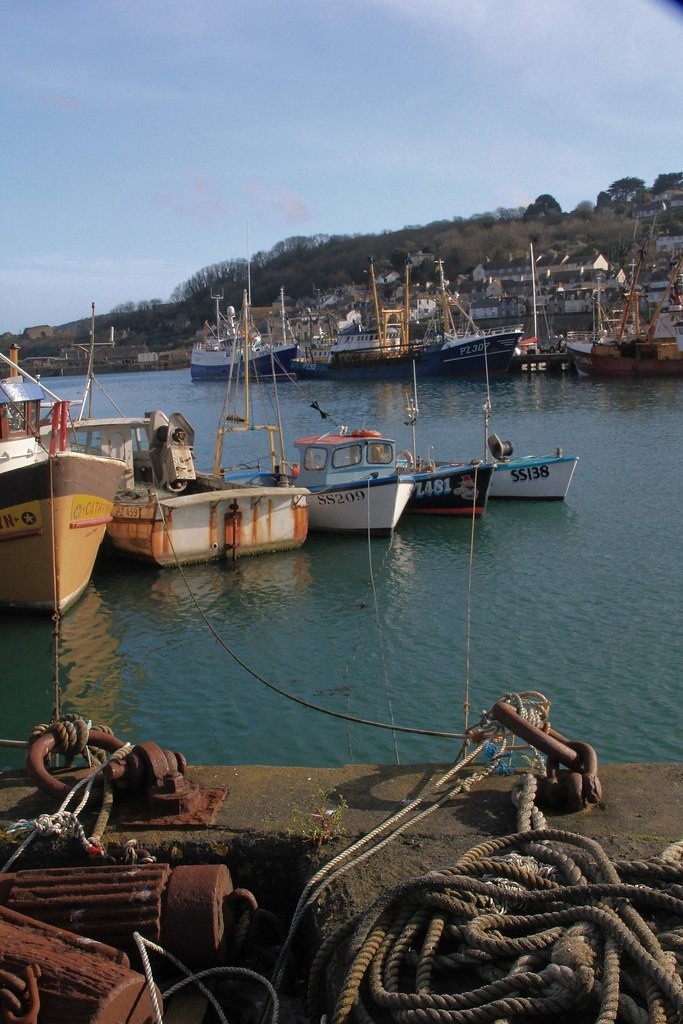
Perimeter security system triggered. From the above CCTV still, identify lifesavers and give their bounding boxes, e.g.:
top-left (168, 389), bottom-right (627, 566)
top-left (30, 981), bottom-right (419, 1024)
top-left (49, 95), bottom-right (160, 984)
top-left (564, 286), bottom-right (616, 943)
top-left (350, 428), bottom-right (382, 438)
top-left (395, 448), bottom-right (413, 474)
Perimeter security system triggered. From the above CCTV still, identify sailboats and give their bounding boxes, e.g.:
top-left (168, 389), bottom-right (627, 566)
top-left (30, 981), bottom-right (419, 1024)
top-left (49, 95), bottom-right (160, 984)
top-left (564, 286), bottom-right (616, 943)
top-left (291, 252), bottom-right (524, 379)
top-left (515, 241), bottom-right (571, 366)
top-left (189, 217), bottom-right (302, 382)
top-left (402, 359), bottom-right (497, 517)
top-left (476, 332), bottom-right (581, 500)
top-left (566, 202), bottom-right (683, 375)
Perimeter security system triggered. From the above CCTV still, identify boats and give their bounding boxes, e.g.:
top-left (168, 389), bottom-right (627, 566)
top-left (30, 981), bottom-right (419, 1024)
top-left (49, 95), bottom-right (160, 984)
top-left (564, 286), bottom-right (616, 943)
top-left (225, 425), bottom-right (414, 537)
top-left (0, 375), bottom-right (128, 619)
top-left (43, 297), bottom-right (310, 571)
